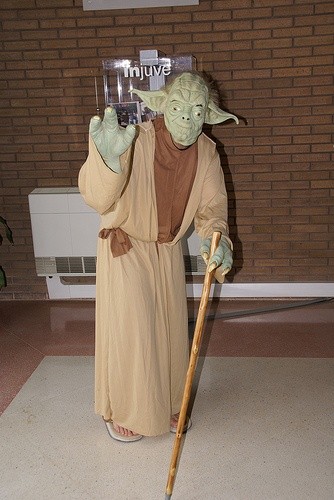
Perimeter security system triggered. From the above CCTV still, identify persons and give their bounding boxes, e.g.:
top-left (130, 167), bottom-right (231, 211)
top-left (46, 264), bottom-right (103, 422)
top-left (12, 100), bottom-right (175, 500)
top-left (76, 70), bottom-right (241, 443)
